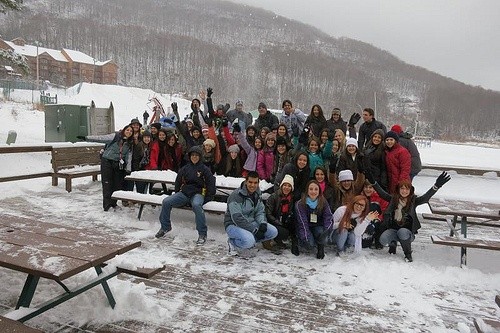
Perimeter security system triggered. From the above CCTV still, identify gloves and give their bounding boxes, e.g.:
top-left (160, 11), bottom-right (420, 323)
top-left (207, 88), bottom-right (213, 98)
top-left (364, 144), bottom-right (376, 156)
top-left (434, 171), bottom-right (451, 189)
top-left (348, 113), bottom-right (361, 127)
top-left (122, 160), bottom-right (127, 166)
top-left (171, 102), bottom-right (242, 132)
top-left (291, 122), bottom-right (299, 137)
top-left (363, 169), bottom-right (375, 185)
top-left (329, 153), bottom-right (339, 173)
top-left (357, 157), bottom-right (366, 174)
top-left (254, 222), bottom-right (329, 245)
top-left (327, 126), bottom-right (336, 141)
top-left (76, 135), bottom-right (86, 141)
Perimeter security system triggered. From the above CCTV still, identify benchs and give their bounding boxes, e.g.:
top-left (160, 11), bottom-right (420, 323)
top-left (52, 146), bottom-right (105, 192)
top-left (431, 235), bottom-right (500, 251)
top-left (112, 196), bottom-right (225, 215)
top-left (103, 255), bottom-right (166, 277)
top-left (154, 187), bottom-right (268, 202)
top-left (423, 214), bottom-right (500, 228)
top-left (0, 314), bottom-right (44, 333)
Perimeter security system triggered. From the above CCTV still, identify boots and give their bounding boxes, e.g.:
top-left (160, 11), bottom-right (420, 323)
top-left (227, 236), bottom-right (412, 263)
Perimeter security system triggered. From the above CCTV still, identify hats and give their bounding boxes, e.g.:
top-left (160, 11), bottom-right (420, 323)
top-left (236, 98), bottom-right (244, 106)
top-left (130, 118), bottom-right (294, 155)
top-left (346, 137), bottom-right (358, 149)
top-left (191, 98), bottom-right (200, 107)
top-left (370, 124), bottom-right (402, 143)
top-left (339, 170), bottom-right (354, 181)
top-left (280, 174), bottom-right (294, 191)
top-left (332, 108), bottom-right (341, 115)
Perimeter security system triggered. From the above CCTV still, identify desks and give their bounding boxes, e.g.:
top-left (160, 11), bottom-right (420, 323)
top-left (124, 175), bottom-right (275, 220)
top-left (0, 212), bottom-right (140, 322)
top-left (428, 197), bottom-right (500, 268)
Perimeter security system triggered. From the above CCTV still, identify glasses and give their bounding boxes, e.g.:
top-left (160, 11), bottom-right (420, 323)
top-left (354, 201), bottom-right (366, 209)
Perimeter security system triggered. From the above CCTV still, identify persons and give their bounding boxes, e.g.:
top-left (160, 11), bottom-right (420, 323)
top-left (264, 174), bottom-right (301, 256)
top-left (156, 146), bottom-right (216, 245)
top-left (327, 194), bottom-right (380, 257)
top-left (362, 169), bottom-right (452, 262)
top-left (224, 171), bottom-right (278, 260)
top-left (292, 180), bottom-right (334, 259)
top-left (75, 87), bottom-right (423, 213)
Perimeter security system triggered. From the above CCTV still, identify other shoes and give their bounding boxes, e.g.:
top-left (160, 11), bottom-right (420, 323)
top-left (155, 227), bottom-right (172, 238)
top-left (197, 234), bottom-right (207, 245)
top-left (114, 204), bottom-right (122, 210)
